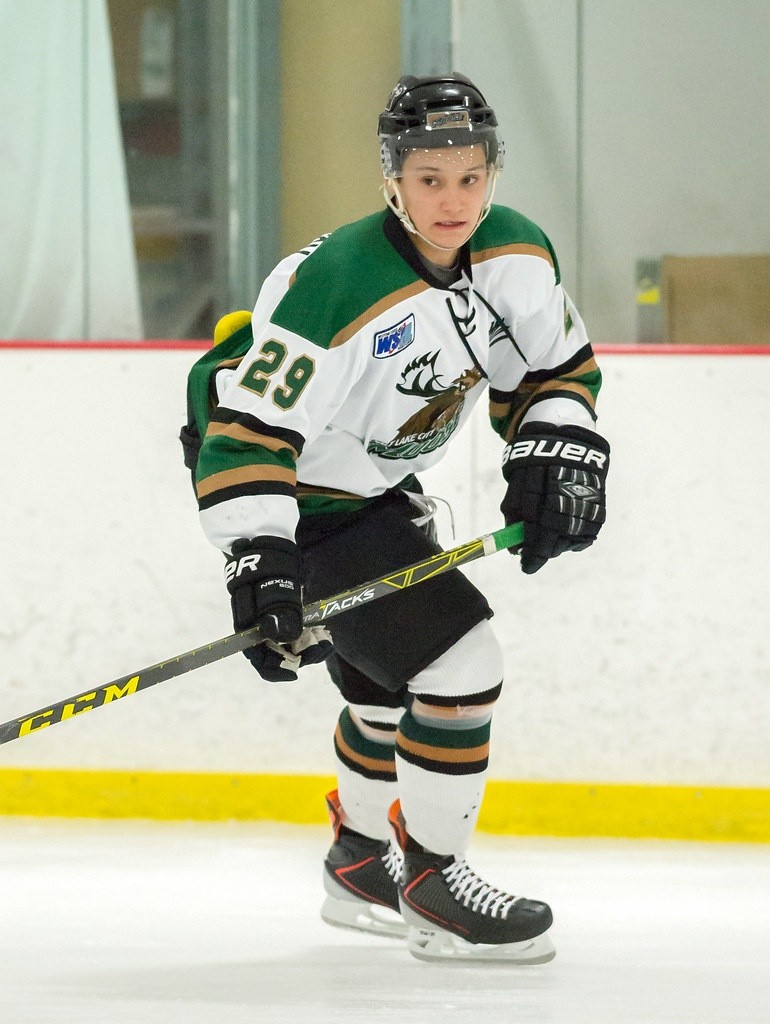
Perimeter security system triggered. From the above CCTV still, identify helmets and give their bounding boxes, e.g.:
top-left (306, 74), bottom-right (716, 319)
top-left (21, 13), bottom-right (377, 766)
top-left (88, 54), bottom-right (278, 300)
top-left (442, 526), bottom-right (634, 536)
top-left (376, 71), bottom-right (506, 179)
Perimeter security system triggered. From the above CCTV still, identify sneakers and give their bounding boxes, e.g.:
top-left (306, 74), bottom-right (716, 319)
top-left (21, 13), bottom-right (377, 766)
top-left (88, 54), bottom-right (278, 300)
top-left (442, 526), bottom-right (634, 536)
top-left (321, 786), bottom-right (405, 937)
top-left (387, 797), bottom-right (555, 960)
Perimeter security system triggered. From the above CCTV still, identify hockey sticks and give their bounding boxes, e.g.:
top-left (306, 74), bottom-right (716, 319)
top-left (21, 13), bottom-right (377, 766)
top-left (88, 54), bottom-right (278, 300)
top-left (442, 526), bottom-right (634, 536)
top-left (0, 522), bottom-right (523, 748)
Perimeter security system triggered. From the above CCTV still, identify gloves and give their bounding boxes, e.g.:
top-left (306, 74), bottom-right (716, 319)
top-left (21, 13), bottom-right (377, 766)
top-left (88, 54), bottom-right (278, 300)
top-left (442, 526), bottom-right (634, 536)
top-left (500, 421), bottom-right (611, 574)
top-left (221, 535), bottom-right (336, 683)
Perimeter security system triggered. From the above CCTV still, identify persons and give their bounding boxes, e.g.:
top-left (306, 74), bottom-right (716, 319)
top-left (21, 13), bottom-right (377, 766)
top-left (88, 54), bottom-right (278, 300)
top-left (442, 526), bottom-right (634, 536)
top-left (179, 69), bottom-right (611, 964)
